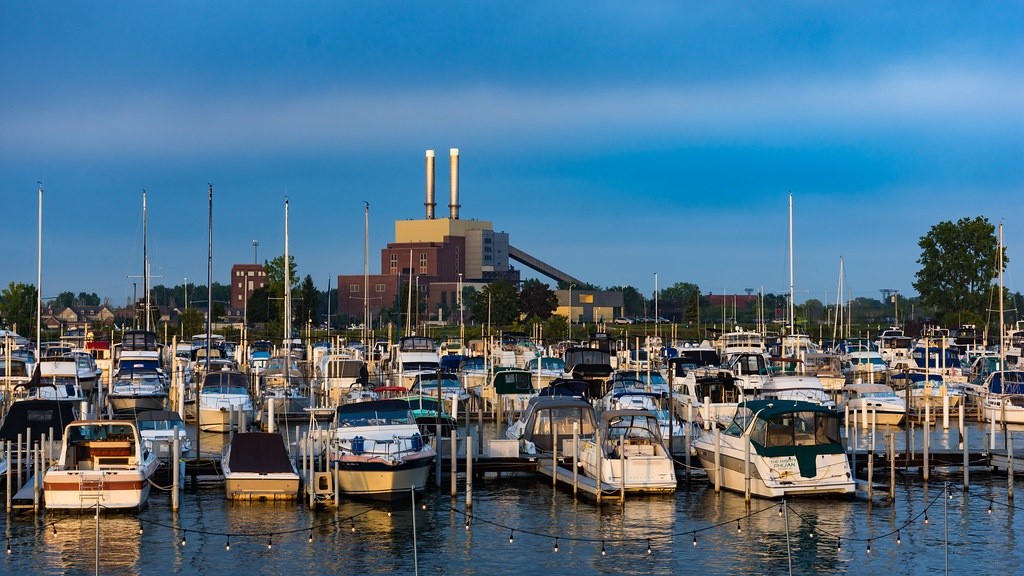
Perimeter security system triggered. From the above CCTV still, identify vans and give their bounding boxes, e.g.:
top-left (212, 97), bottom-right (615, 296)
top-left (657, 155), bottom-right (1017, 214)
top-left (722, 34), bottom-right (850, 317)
top-left (793, 317), bottom-right (809, 324)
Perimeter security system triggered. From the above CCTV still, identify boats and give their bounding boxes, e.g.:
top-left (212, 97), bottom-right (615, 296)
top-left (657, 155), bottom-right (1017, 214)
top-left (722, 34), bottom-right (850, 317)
top-left (218, 424), bottom-right (303, 503)
top-left (42, 410), bottom-right (192, 517)
top-left (469, 329), bottom-right (717, 497)
top-left (671, 368), bottom-right (1024, 503)
top-left (326, 383), bottom-right (462, 503)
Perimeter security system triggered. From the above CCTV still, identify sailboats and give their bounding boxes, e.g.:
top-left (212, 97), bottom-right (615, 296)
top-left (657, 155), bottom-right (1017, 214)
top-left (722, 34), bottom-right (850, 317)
top-left (718, 190), bottom-right (958, 388)
top-left (0, 179), bottom-right (488, 433)
top-left (957, 215), bottom-right (1024, 424)
top-left (651, 272), bottom-right (666, 346)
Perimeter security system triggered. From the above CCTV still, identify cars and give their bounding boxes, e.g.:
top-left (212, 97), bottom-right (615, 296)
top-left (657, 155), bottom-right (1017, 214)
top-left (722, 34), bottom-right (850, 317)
top-left (614, 316), bottom-right (670, 325)
top-left (771, 317), bottom-right (787, 324)
